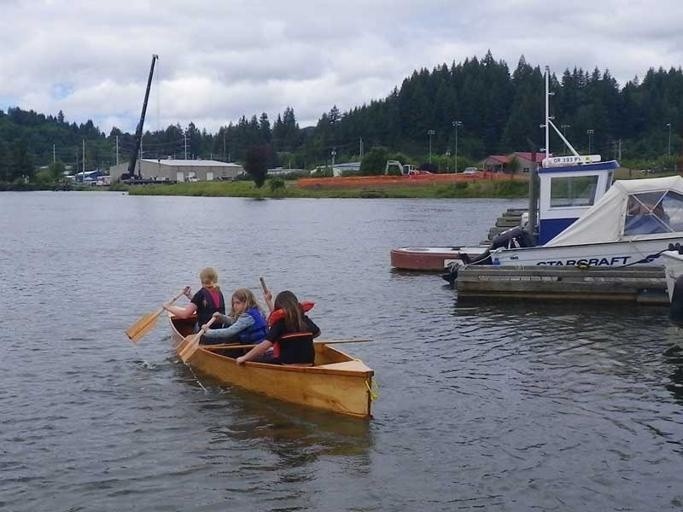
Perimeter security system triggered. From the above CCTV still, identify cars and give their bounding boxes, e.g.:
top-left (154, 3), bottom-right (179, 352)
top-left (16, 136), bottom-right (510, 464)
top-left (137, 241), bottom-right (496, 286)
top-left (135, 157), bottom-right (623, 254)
top-left (464, 167), bottom-right (478, 174)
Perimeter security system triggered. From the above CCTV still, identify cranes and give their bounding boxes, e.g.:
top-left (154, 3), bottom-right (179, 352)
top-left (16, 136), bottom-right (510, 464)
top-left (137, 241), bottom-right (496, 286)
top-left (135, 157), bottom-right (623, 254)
top-left (119, 55), bottom-right (176, 184)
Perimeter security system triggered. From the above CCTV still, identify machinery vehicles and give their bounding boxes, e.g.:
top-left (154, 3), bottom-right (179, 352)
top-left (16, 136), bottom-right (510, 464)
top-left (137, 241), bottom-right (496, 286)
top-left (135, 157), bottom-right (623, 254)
top-left (385, 160), bottom-right (419, 176)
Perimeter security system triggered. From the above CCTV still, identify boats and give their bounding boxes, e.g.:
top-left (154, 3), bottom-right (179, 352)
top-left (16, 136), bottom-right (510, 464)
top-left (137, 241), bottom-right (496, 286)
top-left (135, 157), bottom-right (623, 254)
top-left (391, 69), bottom-right (683, 326)
top-left (167, 306), bottom-right (374, 418)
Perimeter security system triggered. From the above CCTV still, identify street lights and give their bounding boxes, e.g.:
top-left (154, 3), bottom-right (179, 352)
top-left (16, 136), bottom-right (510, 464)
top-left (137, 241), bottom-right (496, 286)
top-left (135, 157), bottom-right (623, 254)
top-left (452, 121), bottom-right (462, 172)
top-left (427, 129), bottom-right (435, 166)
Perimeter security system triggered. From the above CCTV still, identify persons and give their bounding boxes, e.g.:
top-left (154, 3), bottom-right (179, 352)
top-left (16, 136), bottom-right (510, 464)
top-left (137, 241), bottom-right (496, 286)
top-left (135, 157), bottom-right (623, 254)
top-left (201, 287), bottom-right (273, 362)
top-left (236, 290), bottom-right (322, 366)
top-left (162, 266), bottom-right (226, 333)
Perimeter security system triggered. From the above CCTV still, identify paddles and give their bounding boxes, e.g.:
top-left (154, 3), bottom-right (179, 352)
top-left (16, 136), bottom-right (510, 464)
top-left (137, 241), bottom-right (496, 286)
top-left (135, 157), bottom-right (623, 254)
top-left (201, 340), bottom-right (373, 349)
top-left (175, 317), bottom-right (216, 364)
top-left (123, 289), bottom-right (189, 343)
